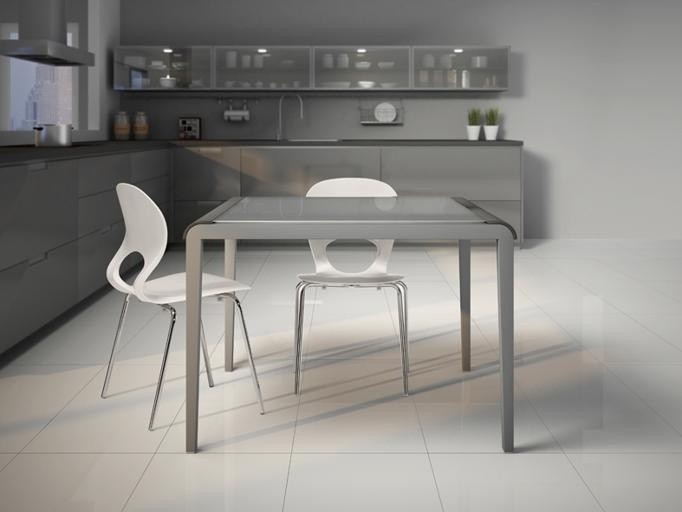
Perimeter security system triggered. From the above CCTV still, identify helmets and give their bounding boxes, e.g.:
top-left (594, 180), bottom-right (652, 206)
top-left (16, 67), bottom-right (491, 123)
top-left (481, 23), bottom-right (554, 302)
top-left (374, 102), bottom-right (397, 122)
top-left (322, 52), bottom-right (396, 88)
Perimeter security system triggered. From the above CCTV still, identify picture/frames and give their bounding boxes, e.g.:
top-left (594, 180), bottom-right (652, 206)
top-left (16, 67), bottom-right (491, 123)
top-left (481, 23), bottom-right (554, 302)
top-left (181, 192), bottom-right (519, 454)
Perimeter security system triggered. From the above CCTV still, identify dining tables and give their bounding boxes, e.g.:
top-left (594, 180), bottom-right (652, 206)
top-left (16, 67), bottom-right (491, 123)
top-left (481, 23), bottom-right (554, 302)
top-left (482, 107), bottom-right (501, 140)
top-left (464, 106), bottom-right (482, 141)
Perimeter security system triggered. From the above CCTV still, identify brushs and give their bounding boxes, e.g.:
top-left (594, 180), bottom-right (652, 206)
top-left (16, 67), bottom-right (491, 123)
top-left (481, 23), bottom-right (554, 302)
top-left (178, 117), bottom-right (200, 140)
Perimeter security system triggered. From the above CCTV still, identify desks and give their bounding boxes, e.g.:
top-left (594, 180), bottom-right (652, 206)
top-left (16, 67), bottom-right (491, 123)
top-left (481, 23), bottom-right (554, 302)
top-left (181, 192), bottom-right (519, 454)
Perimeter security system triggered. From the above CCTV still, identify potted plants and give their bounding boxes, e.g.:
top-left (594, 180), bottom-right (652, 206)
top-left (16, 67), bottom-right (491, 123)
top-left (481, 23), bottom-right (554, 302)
top-left (482, 107), bottom-right (501, 140)
top-left (464, 106), bottom-right (482, 141)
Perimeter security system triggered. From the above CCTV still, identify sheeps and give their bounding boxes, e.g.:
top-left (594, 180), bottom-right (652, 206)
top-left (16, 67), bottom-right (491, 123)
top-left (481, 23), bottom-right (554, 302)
top-left (276, 92), bottom-right (305, 140)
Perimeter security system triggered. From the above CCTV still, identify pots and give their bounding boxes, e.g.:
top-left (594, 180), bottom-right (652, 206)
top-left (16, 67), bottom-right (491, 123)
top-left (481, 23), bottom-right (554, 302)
top-left (32, 124), bottom-right (73, 148)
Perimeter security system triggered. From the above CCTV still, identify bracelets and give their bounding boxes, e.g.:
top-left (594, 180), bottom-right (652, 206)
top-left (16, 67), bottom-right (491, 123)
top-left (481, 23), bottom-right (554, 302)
top-left (160, 78), bottom-right (175, 86)
top-left (224, 51), bottom-right (300, 87)
top-left (147, 61), bottom-right (190, 70)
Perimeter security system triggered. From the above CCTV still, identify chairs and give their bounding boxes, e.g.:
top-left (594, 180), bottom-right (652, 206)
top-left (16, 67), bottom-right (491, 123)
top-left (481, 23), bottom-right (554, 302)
top-left (290, 174), bottom-right (415, 398)
top-left (100, 181), bottom-right (269, 434)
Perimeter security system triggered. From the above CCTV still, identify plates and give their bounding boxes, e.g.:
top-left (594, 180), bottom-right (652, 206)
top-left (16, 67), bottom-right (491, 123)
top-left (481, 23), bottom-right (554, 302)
top-left (311, 45), bottom-right (413, 91)
top-left (213, 46), bottom-right (310, 91)
top-left (412, 45), bottom-right (510, 91)
top-left (111, 47), bottom-right (212, 92)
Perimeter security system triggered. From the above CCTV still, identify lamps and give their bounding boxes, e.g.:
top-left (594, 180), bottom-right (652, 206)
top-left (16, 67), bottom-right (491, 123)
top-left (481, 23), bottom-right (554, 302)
top-left (290, 174), bottom-right (415, 398)
top-left (100, 181), bottom-right (269, 434)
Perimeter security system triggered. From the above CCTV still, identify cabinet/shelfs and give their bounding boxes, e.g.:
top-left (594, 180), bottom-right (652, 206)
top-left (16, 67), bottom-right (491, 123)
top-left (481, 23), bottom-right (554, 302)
top-left (114, 111), bottom-right (149, 141)
top-left (419, 53), bottom-right (489, 88)
top-left (169, 134), bottom-right (526, 247)
top-left (111, 47), bottom-right (212, 92)
top-left (412, 45), bottom-right (510, 91)
top-left (0, 135), bottom-right (172, 358)
top-left (311, 45), bottom-right (413, 91)
top-left (213, 46), bottom-right (310, 91)
